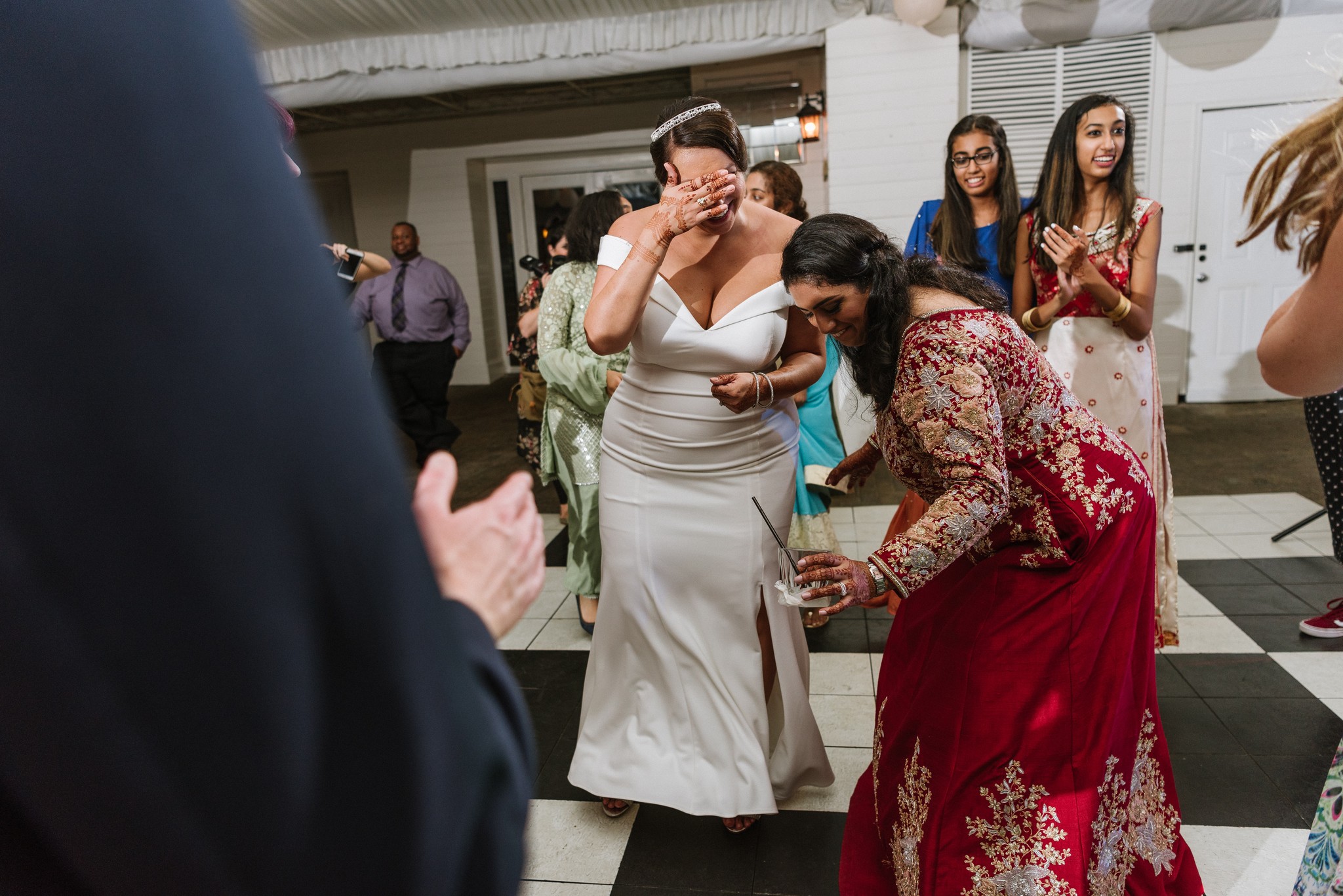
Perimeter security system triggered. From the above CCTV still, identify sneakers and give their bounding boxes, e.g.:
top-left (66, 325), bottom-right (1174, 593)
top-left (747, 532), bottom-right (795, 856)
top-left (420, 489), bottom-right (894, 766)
top-left (1300, 597), bottom-right (1343, 639)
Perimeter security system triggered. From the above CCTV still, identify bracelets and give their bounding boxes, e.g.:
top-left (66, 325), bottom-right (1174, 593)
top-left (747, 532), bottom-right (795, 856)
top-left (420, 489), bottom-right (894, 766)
top-left (751, 372), bottom-right (759, 408)
top-left (1100, 290), bottom-right (1132, 321)
top-left (757, 371), bottom-right (774, 409)
top-left (1022, 307), bottom-right (1052, 332)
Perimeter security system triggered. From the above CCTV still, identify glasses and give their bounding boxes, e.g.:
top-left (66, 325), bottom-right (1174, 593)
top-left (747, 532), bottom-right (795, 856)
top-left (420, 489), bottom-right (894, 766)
top-left (950, 147), bottom-right (1001, 169)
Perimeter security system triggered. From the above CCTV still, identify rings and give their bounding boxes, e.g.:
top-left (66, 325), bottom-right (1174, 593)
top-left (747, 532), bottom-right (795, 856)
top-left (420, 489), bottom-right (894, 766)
top-left (697, 197), bottom-right (706, 209)
top-left (836, 582), bottom-right (847, 597)
top-left (719, 401), bottom-right (725, 407)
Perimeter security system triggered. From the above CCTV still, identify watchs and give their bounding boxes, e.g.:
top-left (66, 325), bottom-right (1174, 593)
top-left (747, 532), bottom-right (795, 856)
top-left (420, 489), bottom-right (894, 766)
top-left (865, 559), bottom-right (885, 597)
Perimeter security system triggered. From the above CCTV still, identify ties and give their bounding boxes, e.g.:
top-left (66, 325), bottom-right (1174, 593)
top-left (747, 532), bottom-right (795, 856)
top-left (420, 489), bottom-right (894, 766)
top-left (391, 263), bottom-right (408, 332)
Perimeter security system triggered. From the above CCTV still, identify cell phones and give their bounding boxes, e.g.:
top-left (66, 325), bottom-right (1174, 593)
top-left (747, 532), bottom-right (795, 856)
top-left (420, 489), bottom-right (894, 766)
top-left (337, 248), bottom-right (365, 282)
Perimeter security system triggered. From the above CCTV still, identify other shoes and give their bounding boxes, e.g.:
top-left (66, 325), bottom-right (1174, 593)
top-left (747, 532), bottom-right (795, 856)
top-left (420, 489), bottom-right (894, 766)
top-left (574, 594), bottom-right (596, 636)
top-left (801, 608), bottom-right (829, 628)
top-left (722, 816), bottom-right (761, 833)
top-left (599, 797), bottom-right (633, 817)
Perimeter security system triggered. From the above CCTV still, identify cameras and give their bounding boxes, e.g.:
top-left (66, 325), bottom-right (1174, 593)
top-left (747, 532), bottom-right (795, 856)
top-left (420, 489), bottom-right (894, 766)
top-left (520, 254), bottom-right (567, 277)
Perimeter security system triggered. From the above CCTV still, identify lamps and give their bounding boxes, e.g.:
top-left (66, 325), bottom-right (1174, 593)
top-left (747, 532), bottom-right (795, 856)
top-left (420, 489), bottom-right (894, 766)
top-left (794, 89), bottom-right (828, 144)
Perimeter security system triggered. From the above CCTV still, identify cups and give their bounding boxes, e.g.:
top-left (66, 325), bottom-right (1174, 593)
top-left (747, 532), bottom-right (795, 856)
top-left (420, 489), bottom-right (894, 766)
top-left (777, 546), bottom-right (832, 607)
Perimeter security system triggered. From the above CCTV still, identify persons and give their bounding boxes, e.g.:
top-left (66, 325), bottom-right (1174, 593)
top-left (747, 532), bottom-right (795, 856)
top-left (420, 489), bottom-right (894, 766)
top-left (744, 160), bottom-right (854, 628)
top-left (322, 242), bottom-right (392, 303)
top-left (351, 222), bottom-right (472, 469)
top-left (1236, 83), bottom-right (1343, 896)
top-left (1298, 388), bottom-right (1343, 636)
top-left (567, 96), bottom-right (836, 834)
top-left (778, 212), bottom-right (1208, 896)
top-left (1008, 94), bottom-right (1182, 651)
top-left (900, 114), bottom-right (1035, 339)
top-left (1, 0), bottom-right (548, 896)
top-left (534, 189), bottom-right (633, 633)
top-left (507, 216), bottom-right (570, 525)
